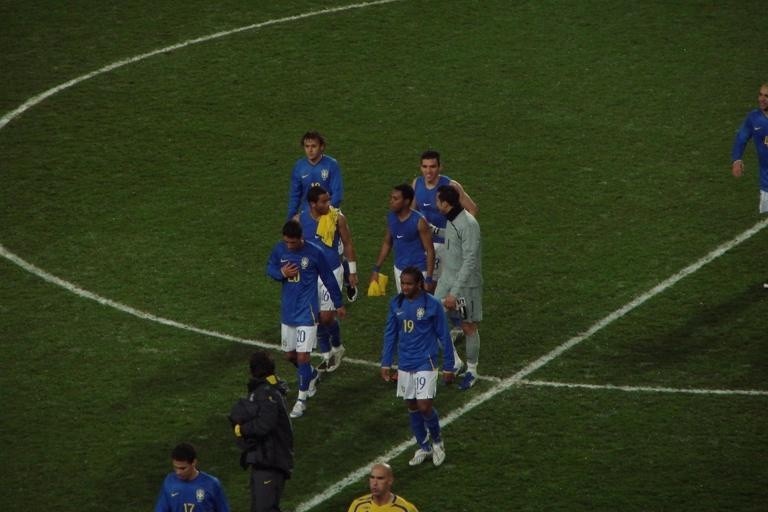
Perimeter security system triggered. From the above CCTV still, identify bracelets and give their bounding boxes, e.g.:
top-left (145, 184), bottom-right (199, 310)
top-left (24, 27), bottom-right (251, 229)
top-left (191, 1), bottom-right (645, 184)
top-left (348, 260), bottom-right (357, 274)
top-left (372, 265), bottom-right (380, 273)
top-left (424, 276), bottom-right (433, 284)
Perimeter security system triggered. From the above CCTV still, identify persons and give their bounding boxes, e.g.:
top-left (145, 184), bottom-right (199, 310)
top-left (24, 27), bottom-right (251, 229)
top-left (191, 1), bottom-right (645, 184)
top-left (379, 264), bottom-right (457, 468)
top-left (290, 185), bottom-right (360, 374)
top-left (154, 442), bottom-right (229, 512)
top-left (428, 184), bottom-right (485, 390)
top-left (230, 350), bottom-right (295, 511)
top-left (731, 82), bottom-right (768, 291)
top-left (286, 130), bottom-right (343, 223)
top-left (409, 150), bottom-right (478, 295)
top-left (369, 182), bottom-right (436, 295)
top-left (265, 220), bottom-right (348, 418)
top-left (346, 462), bottom-right (420, 512)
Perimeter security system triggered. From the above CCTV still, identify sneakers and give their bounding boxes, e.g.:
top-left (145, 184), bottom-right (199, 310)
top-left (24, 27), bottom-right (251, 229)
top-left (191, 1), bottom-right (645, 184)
top-left (457, 372), bottom-right (481, 391)
top-left (307, 369), bottom-right (319, 398)
top-left (431, 440), bottom-right (445, 467)
top-left (326, 345), bottom-right (345, 374)
top-left (347, 284), bottom-right (358, 302)
top-left (409, 447), bottom-right (432, 466)
top-left (450, 326), bottom-right (464, 344)
top-left (440, 361), bottom-right (466, 387)
top-left (290, 399), bottom-right (307, 418)
top-left (391, 372), bottom-right (398, 382)
top-left (315, 359), bottom-right (329, 373)
